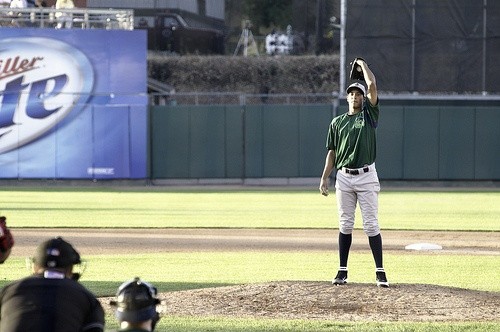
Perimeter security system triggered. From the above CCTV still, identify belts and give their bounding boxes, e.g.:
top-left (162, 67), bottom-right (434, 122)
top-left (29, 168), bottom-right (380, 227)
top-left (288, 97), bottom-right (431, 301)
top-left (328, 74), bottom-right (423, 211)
top-left (346, 167), bottom-right (368, 175)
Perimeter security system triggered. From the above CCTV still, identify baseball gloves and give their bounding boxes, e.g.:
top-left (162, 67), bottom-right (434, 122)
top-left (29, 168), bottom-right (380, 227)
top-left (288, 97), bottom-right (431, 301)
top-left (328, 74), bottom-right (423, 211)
top-left (350, 57), bottom-right (367, 81)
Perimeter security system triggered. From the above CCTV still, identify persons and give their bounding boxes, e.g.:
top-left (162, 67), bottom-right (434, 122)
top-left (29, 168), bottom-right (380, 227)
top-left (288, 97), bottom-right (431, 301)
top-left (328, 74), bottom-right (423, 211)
top-left (0, 236), bottom-right (105, 332)
top-left (319, 57), bottom-right (389, 287)
top-left (8, 0), bottom-right (75, 29)
top-left (110, 277), bottom-right (160, 332)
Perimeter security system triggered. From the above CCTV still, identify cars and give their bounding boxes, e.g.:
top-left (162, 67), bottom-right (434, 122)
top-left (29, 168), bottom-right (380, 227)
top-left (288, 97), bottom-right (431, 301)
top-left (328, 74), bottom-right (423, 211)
top-left (132, 8), bottom-right (225, 55)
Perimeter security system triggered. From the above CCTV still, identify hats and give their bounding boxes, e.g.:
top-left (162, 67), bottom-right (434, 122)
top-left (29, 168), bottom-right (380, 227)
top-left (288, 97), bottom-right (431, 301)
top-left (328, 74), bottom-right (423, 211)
top-left (346, 82), bottom-right (366, 97)
top-left (37, 241), bottom-right (70, 269)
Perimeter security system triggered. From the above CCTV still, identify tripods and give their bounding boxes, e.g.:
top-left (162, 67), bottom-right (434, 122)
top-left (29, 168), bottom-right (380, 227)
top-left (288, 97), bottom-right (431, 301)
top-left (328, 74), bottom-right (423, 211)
top-left (234, 28), bottom-right (259, 58)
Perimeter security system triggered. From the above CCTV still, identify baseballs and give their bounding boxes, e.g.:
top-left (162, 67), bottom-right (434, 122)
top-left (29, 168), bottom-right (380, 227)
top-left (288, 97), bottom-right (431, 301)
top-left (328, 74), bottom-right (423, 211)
top-left (357, 66), bottom-right (362, 72)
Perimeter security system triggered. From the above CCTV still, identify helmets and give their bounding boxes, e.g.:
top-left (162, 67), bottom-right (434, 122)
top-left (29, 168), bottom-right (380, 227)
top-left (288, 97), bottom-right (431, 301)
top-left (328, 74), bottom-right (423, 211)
top-left (116, 278), bottom-right (154, 323)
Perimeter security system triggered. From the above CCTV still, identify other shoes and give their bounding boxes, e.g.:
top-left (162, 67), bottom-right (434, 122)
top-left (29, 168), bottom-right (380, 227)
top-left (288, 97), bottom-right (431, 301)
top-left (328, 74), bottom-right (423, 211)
top-left (376, 269), bottom-right (389, 287)
top-left (332, 267), bottom-right (348, 284)
